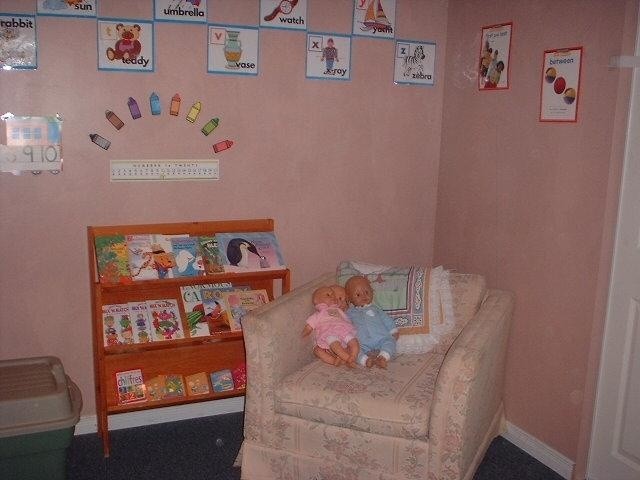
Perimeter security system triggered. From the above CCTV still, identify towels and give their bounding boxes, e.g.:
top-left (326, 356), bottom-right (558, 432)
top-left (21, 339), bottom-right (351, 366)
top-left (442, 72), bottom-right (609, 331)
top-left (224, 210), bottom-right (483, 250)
top-left (336, 260), bottom-right (455, 355)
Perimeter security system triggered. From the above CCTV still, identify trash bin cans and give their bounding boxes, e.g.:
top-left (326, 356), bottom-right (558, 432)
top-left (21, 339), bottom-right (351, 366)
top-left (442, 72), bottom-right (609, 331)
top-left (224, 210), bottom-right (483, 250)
top-left (0, 356), bottom-right (83, 480)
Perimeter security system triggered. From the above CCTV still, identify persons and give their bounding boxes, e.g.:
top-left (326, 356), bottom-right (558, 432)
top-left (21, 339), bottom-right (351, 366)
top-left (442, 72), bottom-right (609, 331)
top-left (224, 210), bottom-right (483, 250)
top-left (301, 285), bottom-right (360, 369)
top-left (309, 283), bottom-right (348, 365)
top-left (342, 276), bottom-right (399, 371)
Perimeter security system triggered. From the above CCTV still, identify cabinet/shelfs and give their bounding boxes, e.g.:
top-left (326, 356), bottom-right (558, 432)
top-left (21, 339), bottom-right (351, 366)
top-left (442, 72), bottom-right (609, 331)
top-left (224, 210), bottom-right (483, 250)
top-left (87, 219), bottom-right (290, 460)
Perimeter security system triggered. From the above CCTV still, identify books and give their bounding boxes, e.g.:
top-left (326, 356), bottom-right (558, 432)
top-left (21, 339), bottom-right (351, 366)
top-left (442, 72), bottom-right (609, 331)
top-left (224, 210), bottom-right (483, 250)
top-left (93, 227), bottom-right (287, 407)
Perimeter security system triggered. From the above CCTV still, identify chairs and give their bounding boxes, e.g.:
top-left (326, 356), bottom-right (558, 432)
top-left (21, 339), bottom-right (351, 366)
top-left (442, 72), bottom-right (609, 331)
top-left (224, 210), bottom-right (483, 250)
top-left (232, 265), bottom-right (513, 480)
